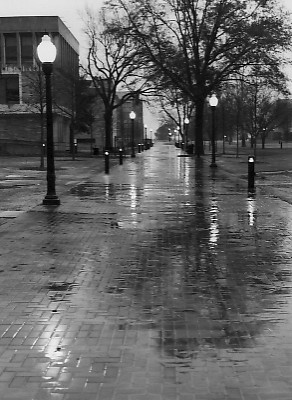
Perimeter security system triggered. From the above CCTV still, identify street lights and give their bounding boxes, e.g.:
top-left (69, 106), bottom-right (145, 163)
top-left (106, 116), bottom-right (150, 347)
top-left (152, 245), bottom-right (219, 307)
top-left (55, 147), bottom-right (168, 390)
top-left (127, 110), bottom-right (137, 156)
top-left (143, 121), bottom-right (181, 149)
top-left (36, 33), bottom-right (60, 207)
top-left (208, 94), bottom-right (216, 168)
top-left (182, 118), bottom-right (190, 155)
